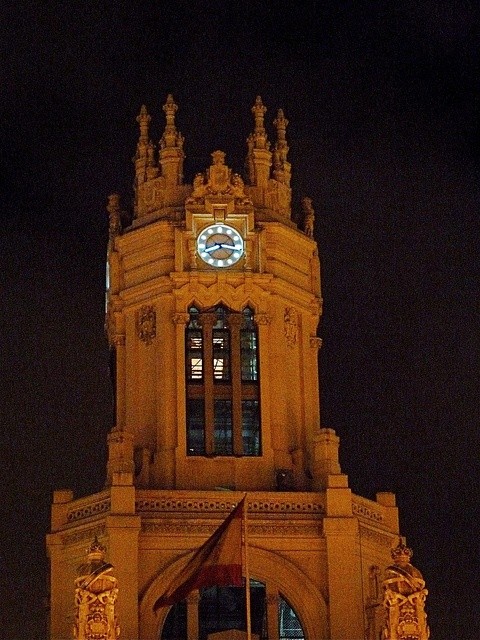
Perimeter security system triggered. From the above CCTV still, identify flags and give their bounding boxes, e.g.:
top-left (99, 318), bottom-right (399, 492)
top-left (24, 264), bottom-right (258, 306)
top-left (153, 494), bottom-right (246, 617)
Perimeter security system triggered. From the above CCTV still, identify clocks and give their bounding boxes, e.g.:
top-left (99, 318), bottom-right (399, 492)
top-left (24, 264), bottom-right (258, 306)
top-left (191, 214), bottom-right (247, 270)
